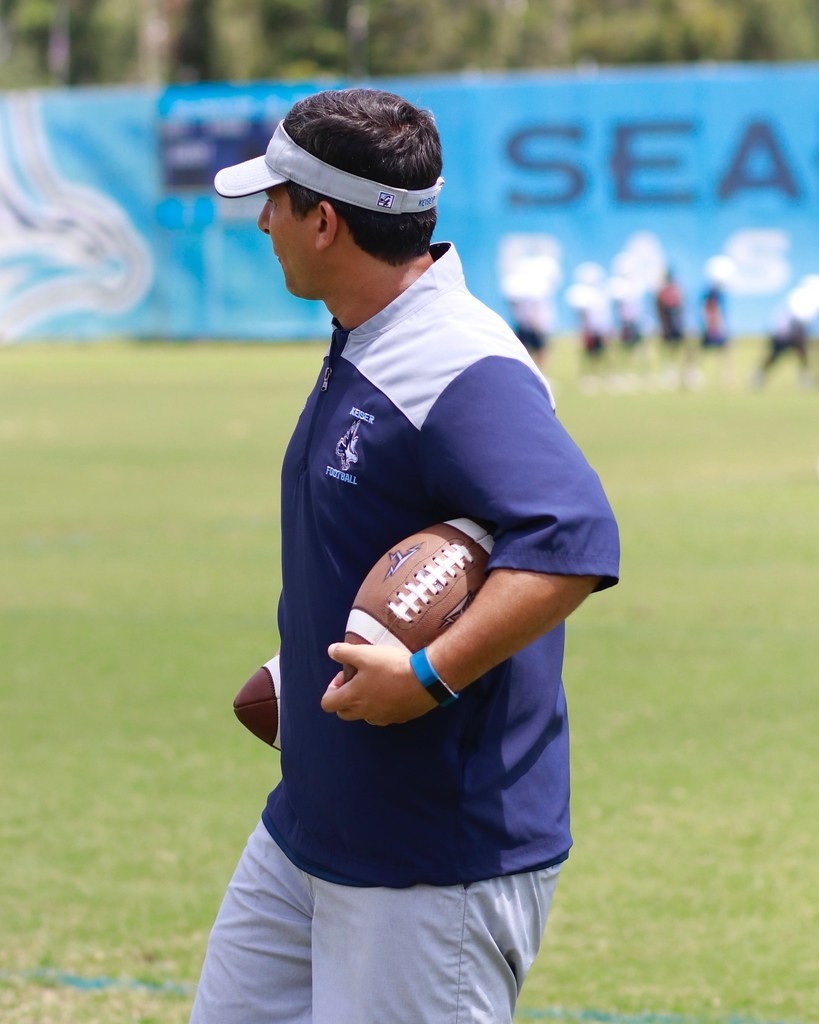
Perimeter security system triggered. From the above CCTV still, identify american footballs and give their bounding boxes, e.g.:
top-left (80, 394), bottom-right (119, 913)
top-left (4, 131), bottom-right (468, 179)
top-left (343, 515), bottom-right (498, 685)
top-left (233, 654), bottom-right (280, 750)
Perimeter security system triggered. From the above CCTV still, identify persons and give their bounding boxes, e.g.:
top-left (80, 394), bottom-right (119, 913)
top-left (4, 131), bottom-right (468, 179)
top-left (511, 260), bottom-right (818, 388)
top-left (189, 88), bottom-right (619, 1024)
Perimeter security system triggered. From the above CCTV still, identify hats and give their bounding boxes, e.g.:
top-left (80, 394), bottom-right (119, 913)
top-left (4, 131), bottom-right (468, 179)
top-left (214, 118), bottom-right (445, 215)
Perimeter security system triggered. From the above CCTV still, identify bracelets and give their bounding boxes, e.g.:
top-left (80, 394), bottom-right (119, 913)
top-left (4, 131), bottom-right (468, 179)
top-left (410, 647), bottom-right (460, 710)
